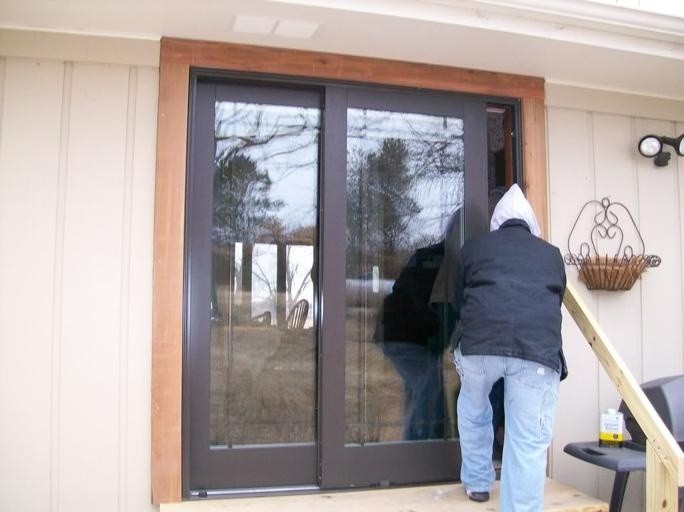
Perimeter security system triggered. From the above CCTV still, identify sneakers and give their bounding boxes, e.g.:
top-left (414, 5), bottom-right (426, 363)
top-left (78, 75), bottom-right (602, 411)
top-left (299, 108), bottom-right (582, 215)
top-left (466, 490), bottom-right (489, 503)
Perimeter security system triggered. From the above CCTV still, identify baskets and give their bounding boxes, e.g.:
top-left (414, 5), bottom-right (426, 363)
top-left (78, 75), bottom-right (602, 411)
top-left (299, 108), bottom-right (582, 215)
top-left (576, 254), bottom-right (650, 290)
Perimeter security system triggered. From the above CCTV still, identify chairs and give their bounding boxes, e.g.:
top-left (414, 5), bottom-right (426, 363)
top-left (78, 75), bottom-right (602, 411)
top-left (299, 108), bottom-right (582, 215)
top-left (252, 311), bottom-right (271, 328)
top-left (261, 298), bottom-right (309, 367)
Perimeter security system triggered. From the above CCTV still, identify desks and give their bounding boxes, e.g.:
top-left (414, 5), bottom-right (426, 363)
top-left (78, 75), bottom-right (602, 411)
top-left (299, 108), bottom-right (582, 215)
top-left (563, 442), bottom-right (646, 511)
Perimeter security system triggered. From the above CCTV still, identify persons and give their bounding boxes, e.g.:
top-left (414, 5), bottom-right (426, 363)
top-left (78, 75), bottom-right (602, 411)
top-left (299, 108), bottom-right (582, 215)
top-left (449, 183), bottom-right (570, 512)
top-left (365, 187), bottom-right (507, 440)
